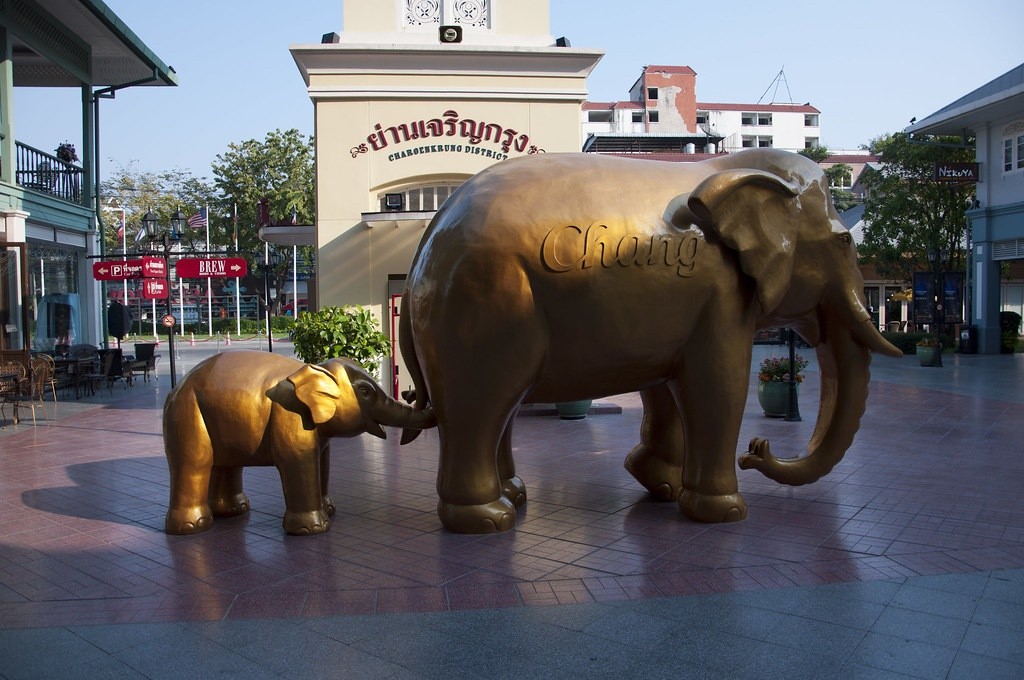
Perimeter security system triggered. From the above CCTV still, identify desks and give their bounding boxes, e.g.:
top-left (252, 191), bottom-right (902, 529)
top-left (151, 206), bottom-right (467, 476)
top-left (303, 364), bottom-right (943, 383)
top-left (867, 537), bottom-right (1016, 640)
top-left (54, 356), bottom-right (96, 400)
top-left (0, 373), bottom-right (23, 425)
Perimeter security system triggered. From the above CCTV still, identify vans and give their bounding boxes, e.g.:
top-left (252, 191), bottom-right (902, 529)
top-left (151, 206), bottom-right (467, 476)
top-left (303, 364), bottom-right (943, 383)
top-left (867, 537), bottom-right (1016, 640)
top-left (156, 305), bottom-right (202, 323)
top-left (275, 299), bottom-right (309, 316)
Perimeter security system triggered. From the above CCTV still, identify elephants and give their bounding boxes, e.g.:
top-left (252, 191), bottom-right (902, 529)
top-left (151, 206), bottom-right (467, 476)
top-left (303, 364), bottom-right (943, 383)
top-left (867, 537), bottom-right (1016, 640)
top-left (161, 352), bottom-right (437, 536)
top-left (398, 148), bottom-right (903, 533)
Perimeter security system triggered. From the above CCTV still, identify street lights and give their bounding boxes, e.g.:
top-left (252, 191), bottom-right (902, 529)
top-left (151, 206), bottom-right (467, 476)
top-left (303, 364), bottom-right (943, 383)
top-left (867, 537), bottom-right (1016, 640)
top-left (142, 203), bottom-right (188, 388)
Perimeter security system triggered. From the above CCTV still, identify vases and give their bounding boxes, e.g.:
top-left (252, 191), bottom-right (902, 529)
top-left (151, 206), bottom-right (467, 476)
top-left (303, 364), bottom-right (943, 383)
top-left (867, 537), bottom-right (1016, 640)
top-left (57, 153), bottom-right (71, 163)
top-left (758, 381), bottom-right (800, 418)
top-left (916, 346), bottom-right (939, 367)
top-left (554, 399), bottom-right (594, 420)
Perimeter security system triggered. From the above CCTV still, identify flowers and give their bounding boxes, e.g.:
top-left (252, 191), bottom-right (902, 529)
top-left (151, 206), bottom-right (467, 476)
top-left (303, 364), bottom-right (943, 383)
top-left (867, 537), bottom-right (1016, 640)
top-left (757, 352), bottom-right (809, 383)
top-left (55, 139), bottom-right (82, 164)
top-left (916, 338), bottom-right (939, 346)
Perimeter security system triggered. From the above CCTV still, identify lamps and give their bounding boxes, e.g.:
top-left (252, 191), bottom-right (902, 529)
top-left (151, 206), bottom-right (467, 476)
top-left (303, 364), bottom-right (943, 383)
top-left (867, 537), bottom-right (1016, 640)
top-left (556, 37), bottom-right (571, 47)
top-left (385, 193), bottom-right (404, 212)
top-left (322, 32), bottom-right (340, 43)
top-left (439, 26), bottom-right (462, 43)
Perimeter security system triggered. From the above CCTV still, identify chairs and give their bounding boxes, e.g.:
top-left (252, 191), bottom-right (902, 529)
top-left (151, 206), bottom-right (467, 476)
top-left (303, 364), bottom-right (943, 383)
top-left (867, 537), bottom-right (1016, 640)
top-left (887, 321), bottom-right (907, 332)
top-left (0, 342), bottom-right (161, 426)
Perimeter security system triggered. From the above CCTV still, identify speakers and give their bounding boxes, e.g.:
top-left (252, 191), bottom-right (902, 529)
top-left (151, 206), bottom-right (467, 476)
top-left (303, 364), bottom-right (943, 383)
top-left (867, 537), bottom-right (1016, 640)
top-left (321, 32), bottom-right (340, 43)
top-left (556, 37), bottom-right (572, 47)
top-left (439, 26), bottom-right (462, 43)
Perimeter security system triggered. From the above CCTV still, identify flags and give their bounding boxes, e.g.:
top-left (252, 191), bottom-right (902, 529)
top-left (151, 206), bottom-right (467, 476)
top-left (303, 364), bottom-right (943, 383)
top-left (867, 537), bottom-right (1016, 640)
top-left (188, 208), bottom-right (207, 228)
top-left (117, 213), bottom-right (124, 238)
